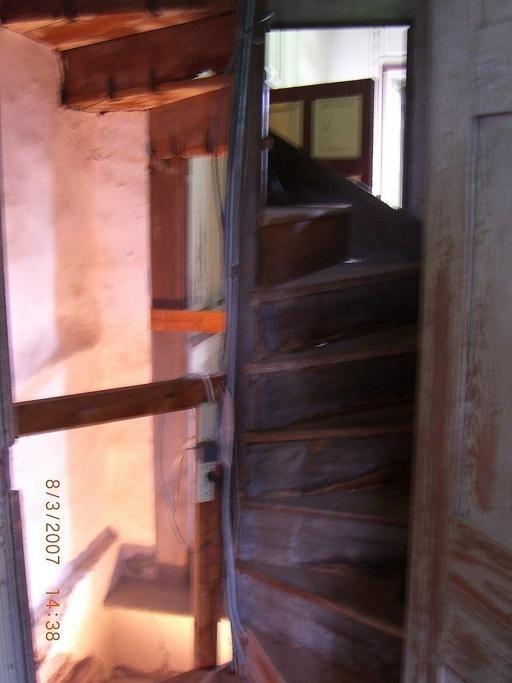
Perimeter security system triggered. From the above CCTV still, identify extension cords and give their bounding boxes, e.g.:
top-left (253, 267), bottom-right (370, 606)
top-left (195, 402), bottom-right (219, 504)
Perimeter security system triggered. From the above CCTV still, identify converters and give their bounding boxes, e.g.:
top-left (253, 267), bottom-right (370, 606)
top-left (196, 441), bottom-right (218, 464)
